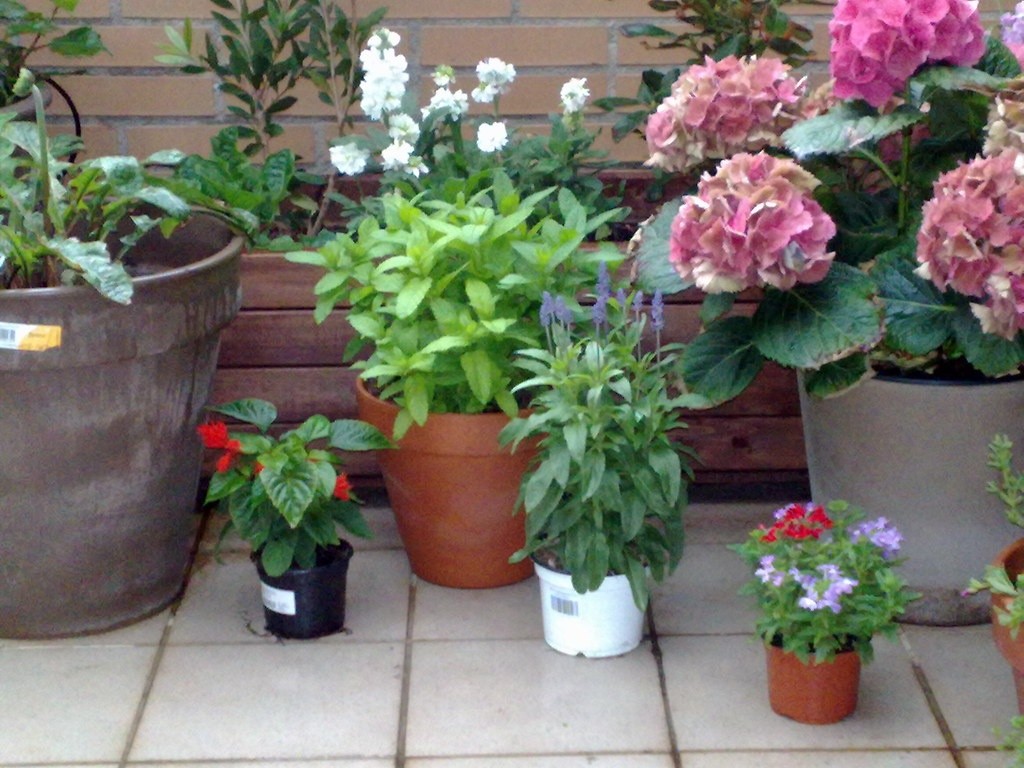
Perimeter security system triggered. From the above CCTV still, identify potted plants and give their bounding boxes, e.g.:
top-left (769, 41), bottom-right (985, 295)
top-left (0, 0), bottom-right (116, 183)
top-left (0, 119), bottom-right (260, 640)
top-left (960, 434), bottom-right (1024, 717)
top-left (284, 167), bottom-right (633, 587)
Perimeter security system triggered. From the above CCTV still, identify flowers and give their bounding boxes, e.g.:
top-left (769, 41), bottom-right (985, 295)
top-left (498, 259), bottom-right (714, 611)
top-left (328, 27), bottom-right (593, 182)
top-left (633, 0), bottom-right (1024, 410)
top-left (197, 398), bottom-right (401, 578)
top-left (724, 497), bottom-right (924, 665)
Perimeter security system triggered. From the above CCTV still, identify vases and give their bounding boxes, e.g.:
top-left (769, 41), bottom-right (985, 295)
top-left (796, 365), bottom-right (1024, 626)
top-left (530, 545), bottom-right (652, 659)
top-left (766, 632), bottom-right (861, 726)
top-left (250, 538), bottom-right (355, 638)
